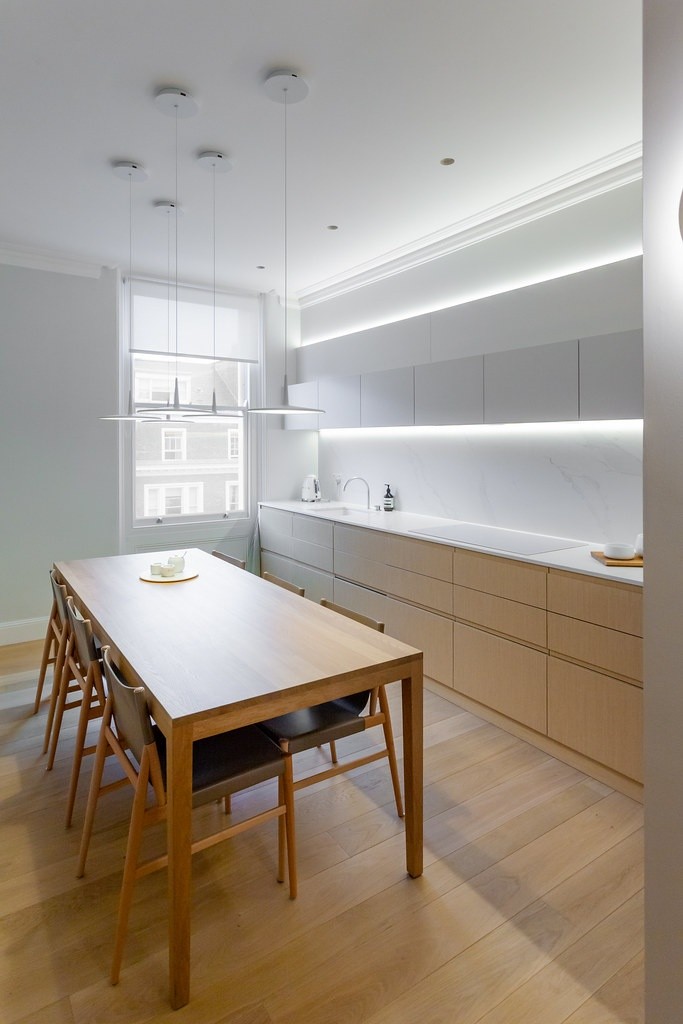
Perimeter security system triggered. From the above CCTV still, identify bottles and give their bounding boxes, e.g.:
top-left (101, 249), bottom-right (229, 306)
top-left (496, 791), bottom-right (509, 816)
top-left (168, 554), bottom-right (185, 572)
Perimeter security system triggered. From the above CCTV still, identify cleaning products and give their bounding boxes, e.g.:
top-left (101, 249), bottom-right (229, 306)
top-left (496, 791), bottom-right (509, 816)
top-left (383, 483), bottom-right (394, 511)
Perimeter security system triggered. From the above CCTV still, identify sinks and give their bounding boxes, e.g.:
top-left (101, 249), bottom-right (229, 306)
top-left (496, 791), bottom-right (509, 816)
top-left (307, 506), bottom-right (370, 512)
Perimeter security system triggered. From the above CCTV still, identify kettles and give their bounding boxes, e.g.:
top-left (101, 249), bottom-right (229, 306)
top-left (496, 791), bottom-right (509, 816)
top-left (301, 473), bottom-right (321, 502)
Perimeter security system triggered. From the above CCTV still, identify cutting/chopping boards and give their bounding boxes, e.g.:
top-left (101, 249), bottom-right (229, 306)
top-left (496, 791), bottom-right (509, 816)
top-left (591, 551), bottom-right (644, 567)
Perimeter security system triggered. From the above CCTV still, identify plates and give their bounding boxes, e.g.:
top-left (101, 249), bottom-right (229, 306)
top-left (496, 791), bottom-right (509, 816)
top-left (139, 567), bottom-right (200, 583)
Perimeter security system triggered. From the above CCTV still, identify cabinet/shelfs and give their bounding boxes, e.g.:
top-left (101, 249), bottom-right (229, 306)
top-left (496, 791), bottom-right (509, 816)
top-left (255, 506), bottom-right (647, 810)
top-left (284, 330), bottom-right (643, 434)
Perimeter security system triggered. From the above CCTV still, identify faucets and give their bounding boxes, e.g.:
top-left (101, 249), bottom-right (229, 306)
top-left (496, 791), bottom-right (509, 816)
top-left (342, 476), bottom-right (370, 509)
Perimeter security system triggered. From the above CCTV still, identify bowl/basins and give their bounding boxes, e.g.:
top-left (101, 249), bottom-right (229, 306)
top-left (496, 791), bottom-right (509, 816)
top-left (603, 542), bottom-right (635, 560)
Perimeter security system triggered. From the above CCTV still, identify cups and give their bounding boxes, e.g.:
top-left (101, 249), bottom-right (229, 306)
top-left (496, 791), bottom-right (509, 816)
top-left (151, 562), bottom-right (164, 575)
top-left (161, 564), bottom-right (175, 577)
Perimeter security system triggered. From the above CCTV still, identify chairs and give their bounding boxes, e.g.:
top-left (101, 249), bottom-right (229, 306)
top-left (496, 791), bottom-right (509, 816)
top-left (34, 551), bottom-right (406, 986)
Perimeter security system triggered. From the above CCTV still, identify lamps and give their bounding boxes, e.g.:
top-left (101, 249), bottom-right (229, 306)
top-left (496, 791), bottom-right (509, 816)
top-left (99, 68), bottom-right (324, 426)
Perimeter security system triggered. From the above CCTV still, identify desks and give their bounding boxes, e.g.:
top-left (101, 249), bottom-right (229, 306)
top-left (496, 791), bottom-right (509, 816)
top-left (53, 548), bottom-right (424, 1012)
